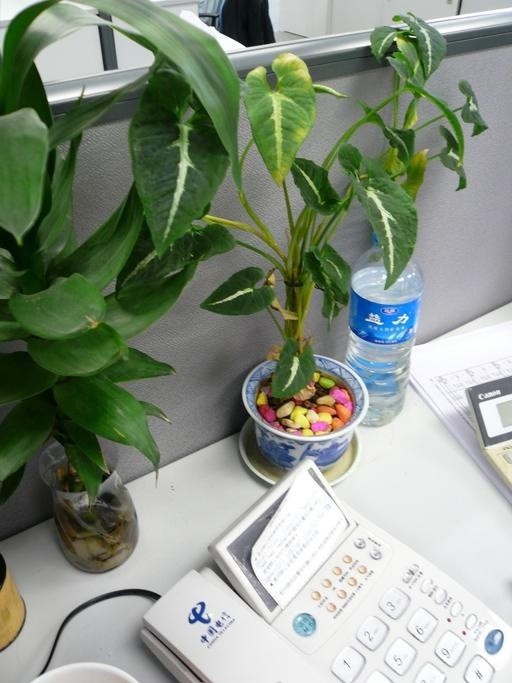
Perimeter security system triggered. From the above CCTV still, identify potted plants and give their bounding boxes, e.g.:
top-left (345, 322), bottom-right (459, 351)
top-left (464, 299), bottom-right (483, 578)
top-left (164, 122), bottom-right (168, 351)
top-left (131, 11), bottom-right (488, 486)
top-left (0, 0), bottom-right (243, 573)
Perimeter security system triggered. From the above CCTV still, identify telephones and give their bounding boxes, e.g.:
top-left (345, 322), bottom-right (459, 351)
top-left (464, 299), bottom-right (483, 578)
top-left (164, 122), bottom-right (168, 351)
top-left (140, 460), bottom-right (512, 683)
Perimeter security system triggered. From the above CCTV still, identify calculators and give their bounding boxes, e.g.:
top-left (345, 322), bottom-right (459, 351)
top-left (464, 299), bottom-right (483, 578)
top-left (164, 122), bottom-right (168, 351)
top-left (465, 375), bottom-right (512, 490)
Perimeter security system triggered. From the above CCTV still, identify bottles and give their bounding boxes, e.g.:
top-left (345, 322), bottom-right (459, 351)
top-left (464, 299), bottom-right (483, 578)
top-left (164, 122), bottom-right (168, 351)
top-left (342, 223), bottom-right (427, 429)
